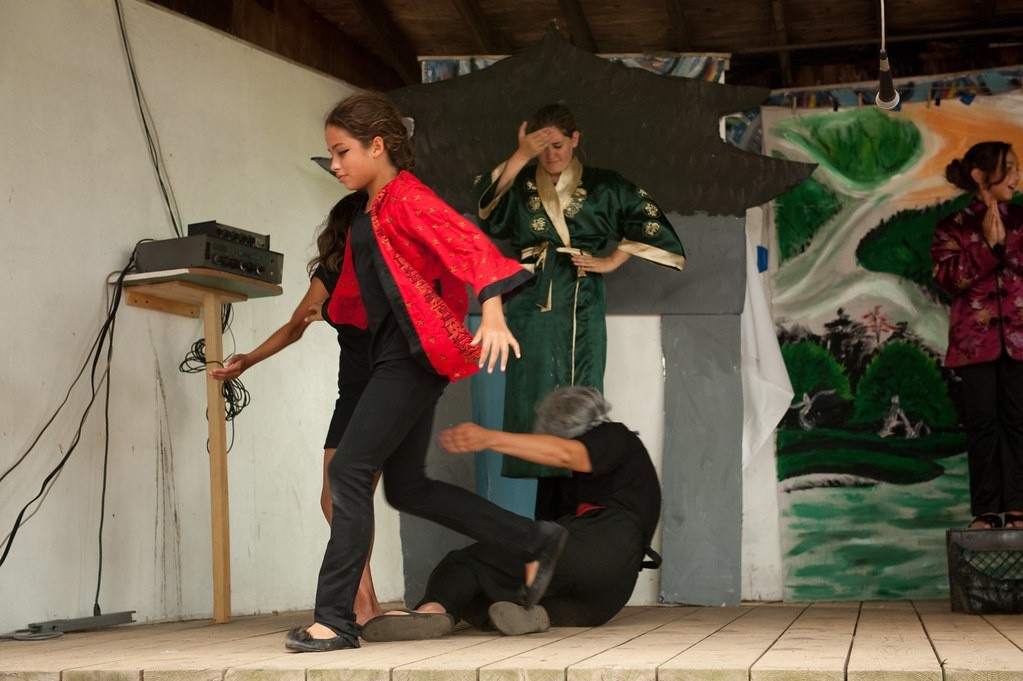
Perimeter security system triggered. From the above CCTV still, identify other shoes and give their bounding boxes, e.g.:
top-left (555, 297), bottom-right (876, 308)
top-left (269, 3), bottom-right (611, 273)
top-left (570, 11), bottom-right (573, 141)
top-left (486, 599), bottom-right (551, 636)
top-left (284, 621), bottom-right (356, 651)
top-left (514, 519), bottom-right (569, 611)
top-left (359, 608), bottom-right (455, 641)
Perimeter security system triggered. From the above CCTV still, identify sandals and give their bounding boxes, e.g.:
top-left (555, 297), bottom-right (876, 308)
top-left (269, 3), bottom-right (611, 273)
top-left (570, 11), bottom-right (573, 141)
top-left (1002, 511), bottom-right (1023, 530)
top-left (967, 513), bottom-right (1003, 529)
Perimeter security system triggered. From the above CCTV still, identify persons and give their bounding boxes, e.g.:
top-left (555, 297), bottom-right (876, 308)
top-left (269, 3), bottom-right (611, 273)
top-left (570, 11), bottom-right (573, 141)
top-left (285, 94), bottom-right (566, 652)
top-left (474, 105), bottom-right (687, 478)
top-left (361, 387), bottom-right (662, 642)
top-left (210, 192), bottom-right (383, 626)
top-left (930, 139), bottom-right (1023, 530)
